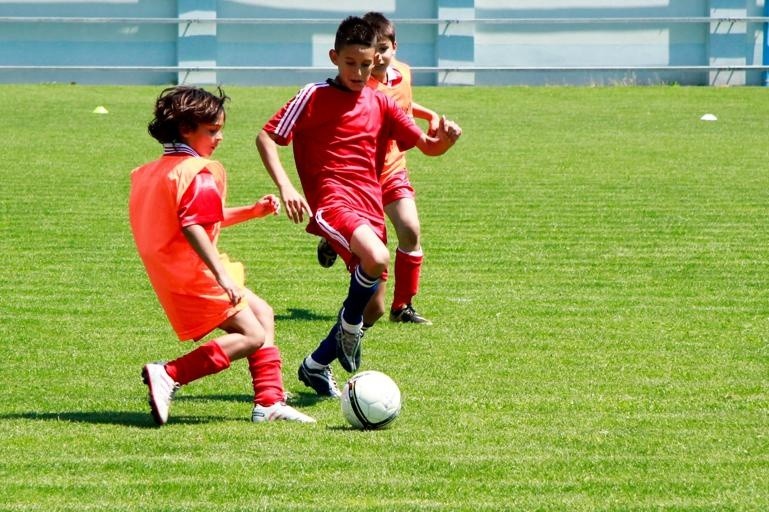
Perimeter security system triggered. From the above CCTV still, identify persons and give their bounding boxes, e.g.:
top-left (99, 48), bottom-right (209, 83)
top-left (255, 14), bottom-right (463, 402)
top-left (314, 12), bottom-right (443, 328)
top-left (125, 81), bottom-right (321, 429)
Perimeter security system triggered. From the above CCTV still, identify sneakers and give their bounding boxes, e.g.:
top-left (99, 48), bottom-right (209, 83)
top-left (298, 358), bottom-right (342, 398)
top-left (251, 402), bottom-right (317, 423)
top-left (390, 303), bottom-right (433, 326)
top-left (141, 364), bottom-right (180, 425)
top-left (335, 307), bottom-right (364, 373)
top-left (318, 237), bottom-right (337, 268)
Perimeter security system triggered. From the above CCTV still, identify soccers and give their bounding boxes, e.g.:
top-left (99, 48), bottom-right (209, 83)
top-left (341, 372), bottom-right (403, 428)
